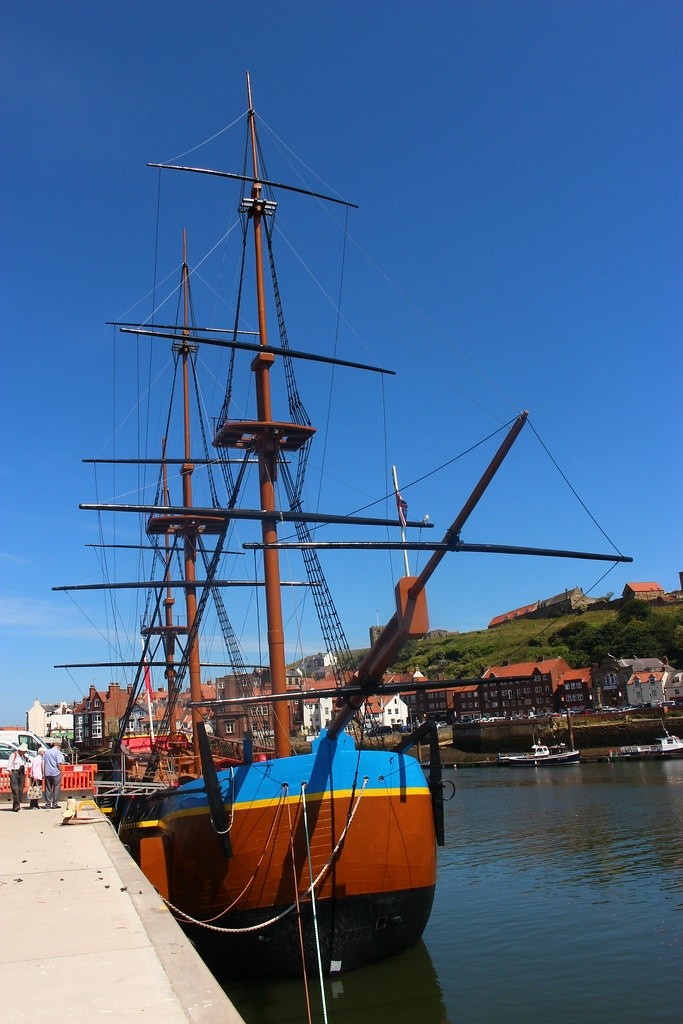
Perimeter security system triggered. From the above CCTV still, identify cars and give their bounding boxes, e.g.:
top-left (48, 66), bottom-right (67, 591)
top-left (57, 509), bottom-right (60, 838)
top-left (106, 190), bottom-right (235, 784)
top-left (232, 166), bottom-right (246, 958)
top-left (0, 740), bottom-right (37, 767)
top-left (435, 699), bottom-right (677, 729)
top-left (398, 726), bottom-right (411, 733)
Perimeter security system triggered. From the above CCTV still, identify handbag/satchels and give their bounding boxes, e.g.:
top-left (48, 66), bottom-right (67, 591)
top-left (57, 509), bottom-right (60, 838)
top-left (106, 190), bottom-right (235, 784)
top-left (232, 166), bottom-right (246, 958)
top-left (27, 780), bottom-right (42, 800)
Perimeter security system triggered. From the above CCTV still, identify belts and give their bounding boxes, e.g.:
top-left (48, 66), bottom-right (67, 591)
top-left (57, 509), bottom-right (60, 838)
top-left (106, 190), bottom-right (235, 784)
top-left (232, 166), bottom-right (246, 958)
top-left (12, 770), bottom-right (19, 771)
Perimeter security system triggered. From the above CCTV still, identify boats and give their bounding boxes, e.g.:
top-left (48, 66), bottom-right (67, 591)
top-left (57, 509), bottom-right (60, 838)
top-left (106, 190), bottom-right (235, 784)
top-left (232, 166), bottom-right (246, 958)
top-left (618, 736), bottom-right (682, 759)
top-left (496, 737), bottom-right (581, 768)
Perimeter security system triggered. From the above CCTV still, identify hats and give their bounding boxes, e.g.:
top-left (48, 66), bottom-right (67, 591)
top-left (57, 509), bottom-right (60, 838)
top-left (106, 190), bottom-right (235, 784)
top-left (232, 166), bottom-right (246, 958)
top-left (54, 743), bottom-right (63, 748)
top-left (17, 743), bottom-right (29, 751)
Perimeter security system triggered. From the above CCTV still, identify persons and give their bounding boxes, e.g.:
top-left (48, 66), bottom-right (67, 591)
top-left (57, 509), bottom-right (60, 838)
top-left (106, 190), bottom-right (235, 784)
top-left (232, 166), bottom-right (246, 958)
top-left (27, 747), bottom-right (45, 810)
top-left (42, 742), bottom-right (63, 809)
top-left (6, 743), bottom-right (28, 811)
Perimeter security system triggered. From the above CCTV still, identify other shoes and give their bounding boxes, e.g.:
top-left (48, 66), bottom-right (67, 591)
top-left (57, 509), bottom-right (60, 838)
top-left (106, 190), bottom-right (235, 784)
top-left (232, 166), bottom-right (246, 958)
top-left (54, 805), bottom-right (61, 808)
top-left (14, 808), bottom-right (21, 812)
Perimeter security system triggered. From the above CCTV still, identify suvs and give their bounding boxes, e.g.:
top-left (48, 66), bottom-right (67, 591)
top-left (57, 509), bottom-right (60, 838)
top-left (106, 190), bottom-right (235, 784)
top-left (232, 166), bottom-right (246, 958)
top-left (365, 726), bottom-right (392, 737)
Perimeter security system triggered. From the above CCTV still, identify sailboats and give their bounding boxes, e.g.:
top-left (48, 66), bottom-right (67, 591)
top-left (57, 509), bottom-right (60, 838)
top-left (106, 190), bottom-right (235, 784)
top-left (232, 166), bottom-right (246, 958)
top-left (51, 71), bottom-right (636, 956)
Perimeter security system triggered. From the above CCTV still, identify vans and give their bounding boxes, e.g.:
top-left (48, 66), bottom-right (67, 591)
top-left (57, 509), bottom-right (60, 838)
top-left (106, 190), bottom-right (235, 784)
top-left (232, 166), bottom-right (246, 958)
top-left (0, 731), bottom-right (52, 756)
